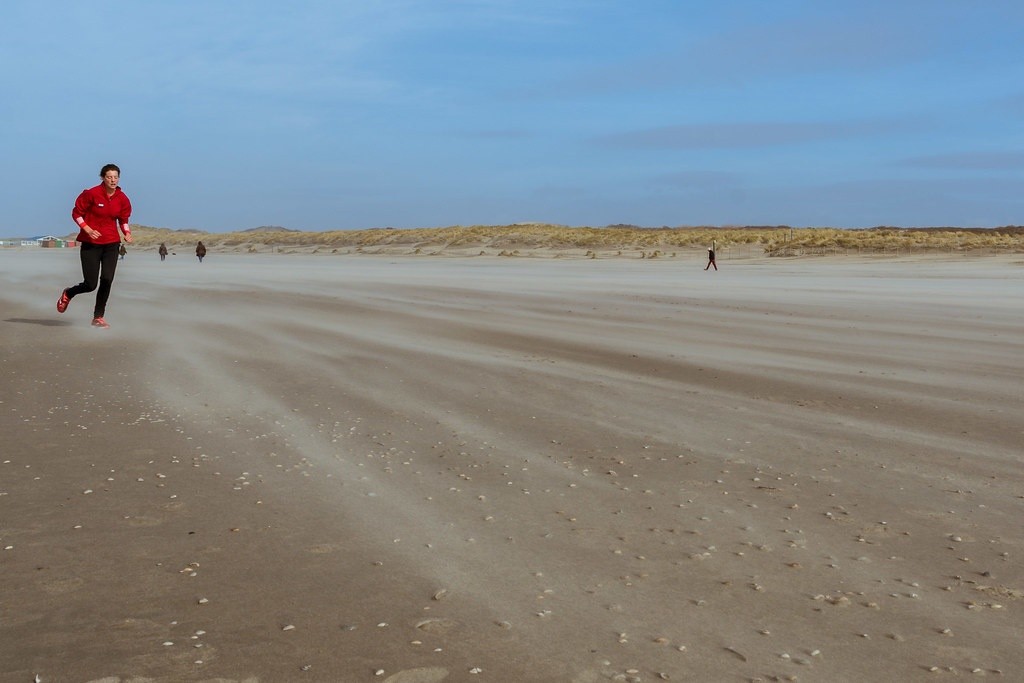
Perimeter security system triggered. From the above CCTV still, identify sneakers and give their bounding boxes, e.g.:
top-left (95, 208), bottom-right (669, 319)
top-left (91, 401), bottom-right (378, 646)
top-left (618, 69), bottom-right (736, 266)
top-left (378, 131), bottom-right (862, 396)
top-left (57, 287), bottom-right (71, 313)
top-left (91, 317), bottom-right (110, 328)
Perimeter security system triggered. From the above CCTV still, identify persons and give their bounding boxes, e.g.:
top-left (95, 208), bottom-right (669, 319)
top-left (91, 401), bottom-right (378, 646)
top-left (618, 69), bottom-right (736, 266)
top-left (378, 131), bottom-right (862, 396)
top-left (158, 244), bottom-right (168, 261)
top-left (57, 163), bottom-right (132, 329)
top-left (196, 241), bottom-right (206, 263)
top-left (704, 247), bottom-right (718, 271)
top-left (119, 243), bottom-right (127, 260)
